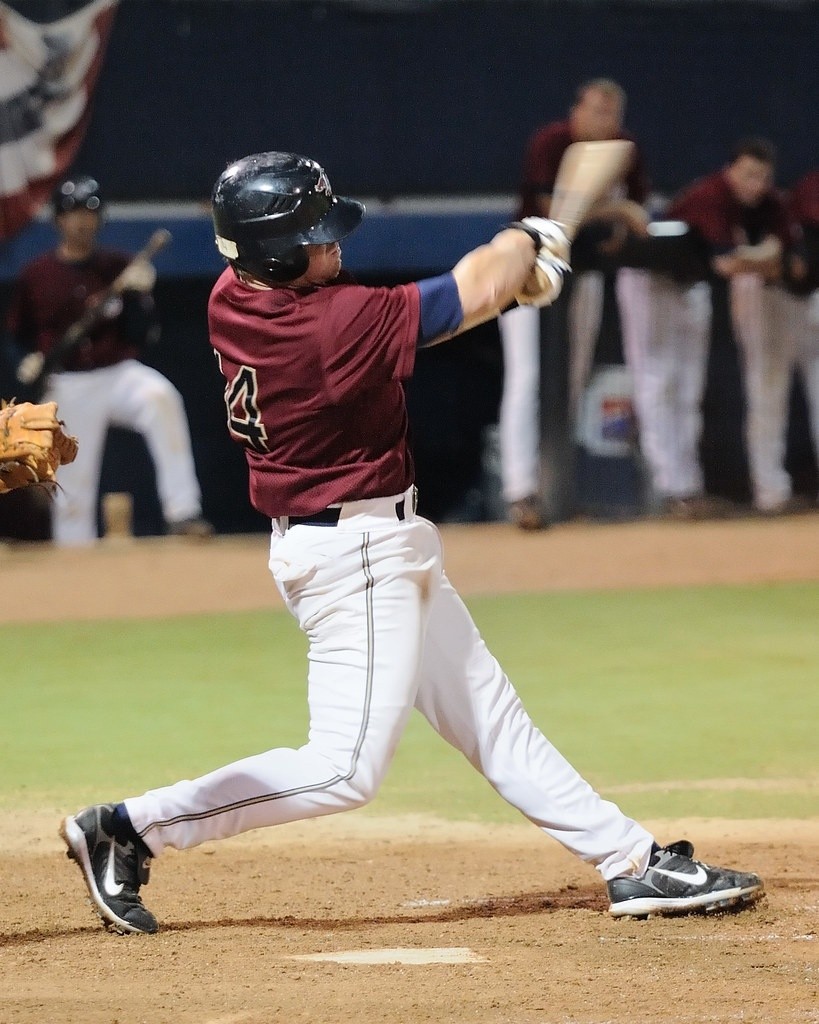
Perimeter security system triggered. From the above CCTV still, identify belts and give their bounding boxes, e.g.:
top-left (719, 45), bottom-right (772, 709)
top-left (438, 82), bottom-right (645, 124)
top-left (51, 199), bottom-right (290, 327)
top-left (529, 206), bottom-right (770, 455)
top-left (287, 502), bottom-right (407, 528)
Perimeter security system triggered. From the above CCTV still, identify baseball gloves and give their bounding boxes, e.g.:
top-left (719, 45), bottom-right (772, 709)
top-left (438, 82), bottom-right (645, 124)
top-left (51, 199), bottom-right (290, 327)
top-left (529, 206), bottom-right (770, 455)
top-left (0, 397), bottom-right (76, 500)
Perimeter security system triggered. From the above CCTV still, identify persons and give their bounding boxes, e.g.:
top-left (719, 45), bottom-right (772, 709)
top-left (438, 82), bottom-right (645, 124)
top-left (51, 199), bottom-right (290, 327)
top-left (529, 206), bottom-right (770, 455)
top-left (60, 152), bottom-right (764, 934)
top-left (496, 79), bottom-right (819, 527)
top-left (10, 174), bottom-right (215, 542)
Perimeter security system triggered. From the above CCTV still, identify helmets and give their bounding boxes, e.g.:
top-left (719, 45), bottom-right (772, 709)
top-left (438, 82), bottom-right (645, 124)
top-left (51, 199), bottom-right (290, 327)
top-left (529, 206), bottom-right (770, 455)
top-left (51, 174), bottom-right (103, 216)
top-left (211, 151), bottom-right (365, 291)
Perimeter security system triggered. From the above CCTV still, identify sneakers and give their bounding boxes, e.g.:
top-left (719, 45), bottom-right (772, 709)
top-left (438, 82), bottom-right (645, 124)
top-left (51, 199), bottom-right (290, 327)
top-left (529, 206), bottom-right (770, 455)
top-left (59, 803), bottom-right (158, 934)
top-left (605, 839), bottom-right (764, 916)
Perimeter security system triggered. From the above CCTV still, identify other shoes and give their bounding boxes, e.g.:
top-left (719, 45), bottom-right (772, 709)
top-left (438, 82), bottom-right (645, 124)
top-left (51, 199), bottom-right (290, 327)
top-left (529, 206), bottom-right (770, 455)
top-left (171, 515), bottom-right (211, 539)
top-left (511, 496), bottom-right (546, 531)
top-left (664, 495), bottom-right (725, 521)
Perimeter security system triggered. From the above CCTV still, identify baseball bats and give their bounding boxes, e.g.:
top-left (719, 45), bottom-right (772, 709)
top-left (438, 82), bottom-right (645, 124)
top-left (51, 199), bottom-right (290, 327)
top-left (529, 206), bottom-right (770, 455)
top-left (522, 133), bottom-right (638, 293)
